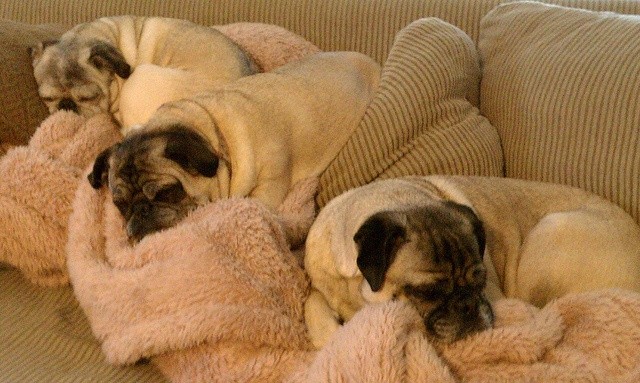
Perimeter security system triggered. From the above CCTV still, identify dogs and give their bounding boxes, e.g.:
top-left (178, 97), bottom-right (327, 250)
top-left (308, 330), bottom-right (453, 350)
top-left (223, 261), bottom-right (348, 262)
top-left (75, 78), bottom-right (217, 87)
top-left (87, 50), bottom-right (382, 247)
top-left (28, 14), bottom-right (267, 138)
top-left (303, 173), bottom-right (639, 348)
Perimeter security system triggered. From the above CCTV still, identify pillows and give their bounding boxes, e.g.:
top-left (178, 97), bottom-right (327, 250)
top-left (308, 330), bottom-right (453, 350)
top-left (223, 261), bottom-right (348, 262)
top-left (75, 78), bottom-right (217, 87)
top-left (0, 20), bottom-right (67, 155)
top-left (315, 16), bottom-right (505, 210)
top-left (477, 2), bottom-right (640, 224)
